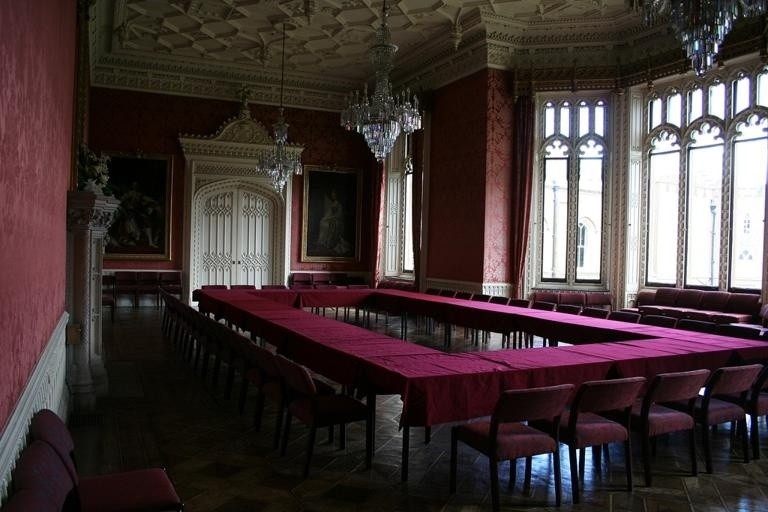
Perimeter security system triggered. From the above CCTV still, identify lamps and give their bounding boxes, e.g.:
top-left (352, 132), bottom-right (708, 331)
top-left (626, 0), bottom-right (766, 81)
top-left (255, 22), bottom-right (303, 194)
top-left (340, 1), bottom-right (426, 164)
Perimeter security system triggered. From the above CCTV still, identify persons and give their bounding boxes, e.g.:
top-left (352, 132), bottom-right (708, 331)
top-left (323, 189), bottom-right (344, 247)
top-left (121, 183), bottom-right (159, 248)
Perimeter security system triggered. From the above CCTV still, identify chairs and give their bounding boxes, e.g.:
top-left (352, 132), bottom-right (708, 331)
top-left (31, 408), bottom-right (185, 508)
top-left (102, 271), bottom-right (184, 325)
top-left (9, 442), bottom-right (183, 512)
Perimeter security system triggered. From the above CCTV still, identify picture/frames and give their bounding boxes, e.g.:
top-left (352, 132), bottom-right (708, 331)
top-left (301, 164), bottom-right (364, 264)
top-left (102, 152), bottom-right (174, 262)
